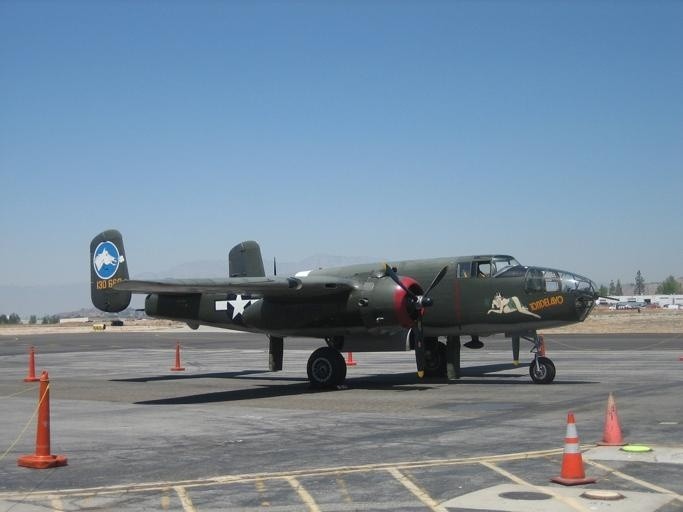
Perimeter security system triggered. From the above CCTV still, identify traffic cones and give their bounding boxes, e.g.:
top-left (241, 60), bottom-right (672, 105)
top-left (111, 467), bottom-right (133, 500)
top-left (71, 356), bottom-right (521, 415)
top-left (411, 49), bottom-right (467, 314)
top-left (549, 411), bottom-right (595, 487)
top-left (594, 392), bottom-right (626, 446)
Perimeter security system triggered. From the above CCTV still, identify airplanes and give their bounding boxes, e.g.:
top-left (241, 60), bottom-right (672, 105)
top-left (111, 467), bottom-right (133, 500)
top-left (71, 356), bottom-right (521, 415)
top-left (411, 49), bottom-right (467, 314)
top-left (90, 229), bottom-right (600, 389)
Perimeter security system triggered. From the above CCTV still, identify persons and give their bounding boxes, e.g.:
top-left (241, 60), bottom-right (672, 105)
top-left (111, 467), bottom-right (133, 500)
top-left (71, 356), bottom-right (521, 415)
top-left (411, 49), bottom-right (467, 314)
top-left (486, 291), bottom-right (541, 320)
